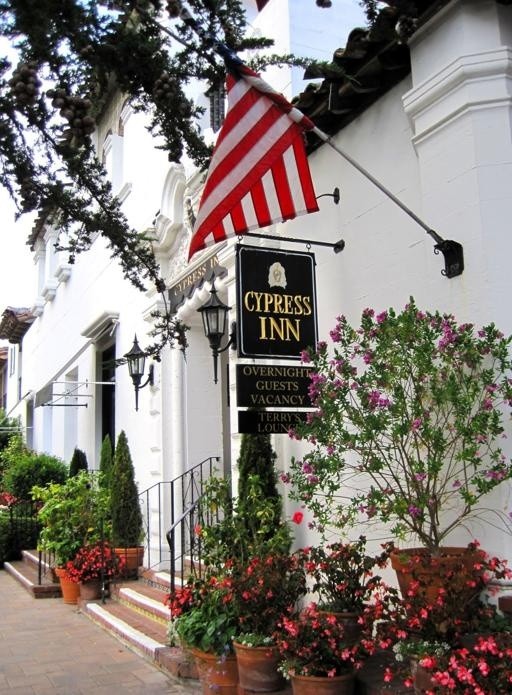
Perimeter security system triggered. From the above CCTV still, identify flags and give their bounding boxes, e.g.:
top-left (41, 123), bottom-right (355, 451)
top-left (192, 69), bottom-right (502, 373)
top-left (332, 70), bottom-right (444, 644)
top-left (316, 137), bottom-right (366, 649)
top-left (172, 7), bottom-right (336, 264)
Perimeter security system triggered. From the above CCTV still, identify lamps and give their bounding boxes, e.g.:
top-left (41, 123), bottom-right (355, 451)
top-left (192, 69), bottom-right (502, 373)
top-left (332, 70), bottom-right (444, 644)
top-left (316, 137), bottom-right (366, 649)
top-left (123, 332), bottom-right (154, 412)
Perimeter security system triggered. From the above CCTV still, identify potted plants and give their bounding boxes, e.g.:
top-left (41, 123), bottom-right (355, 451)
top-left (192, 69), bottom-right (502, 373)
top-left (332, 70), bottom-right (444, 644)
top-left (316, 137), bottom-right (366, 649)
top-left (28, 430), bottom-right (144, 604)
